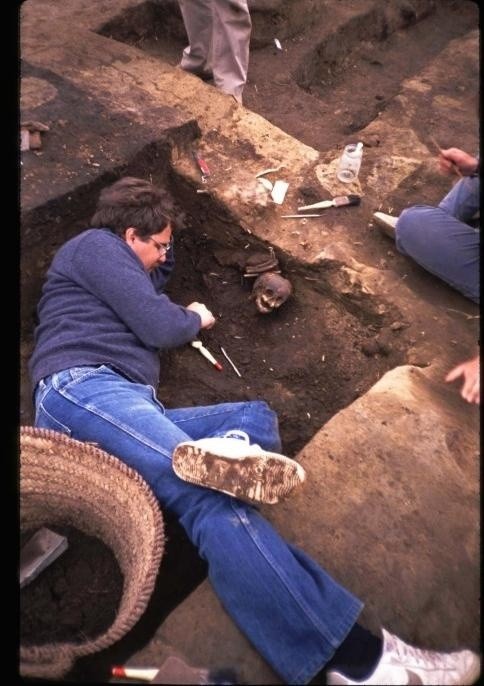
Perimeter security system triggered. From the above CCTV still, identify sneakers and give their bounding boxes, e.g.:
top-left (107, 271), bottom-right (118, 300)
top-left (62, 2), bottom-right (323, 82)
top-left (170, 435), bottom-right (308, 506)
top-left (324, 626), bottom-right (481, 686)
top-left (372, 210), bottom-right (399, 240)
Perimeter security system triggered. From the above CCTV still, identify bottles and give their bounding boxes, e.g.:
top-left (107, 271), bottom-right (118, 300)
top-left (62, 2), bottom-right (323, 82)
top-left (336, 143), bottom-right (364, 183)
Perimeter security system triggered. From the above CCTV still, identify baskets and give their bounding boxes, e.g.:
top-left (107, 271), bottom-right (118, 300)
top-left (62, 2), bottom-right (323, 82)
top-left (19, 423), bottom-right (165, 680)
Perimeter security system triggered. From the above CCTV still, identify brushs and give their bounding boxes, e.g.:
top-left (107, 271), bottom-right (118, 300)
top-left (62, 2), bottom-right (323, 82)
top-left (296, 194), bottom-right (361, 212)
top-left (188, 337), bottom-right (223, 372)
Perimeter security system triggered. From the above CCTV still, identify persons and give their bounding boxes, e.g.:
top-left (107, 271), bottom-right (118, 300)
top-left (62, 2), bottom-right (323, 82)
top-left (27, 175), bottom-right (481, 686)
top-left (370, 145), bottom-right (481, 403)
top-left (173, 0), bottom-right (254, 106)
